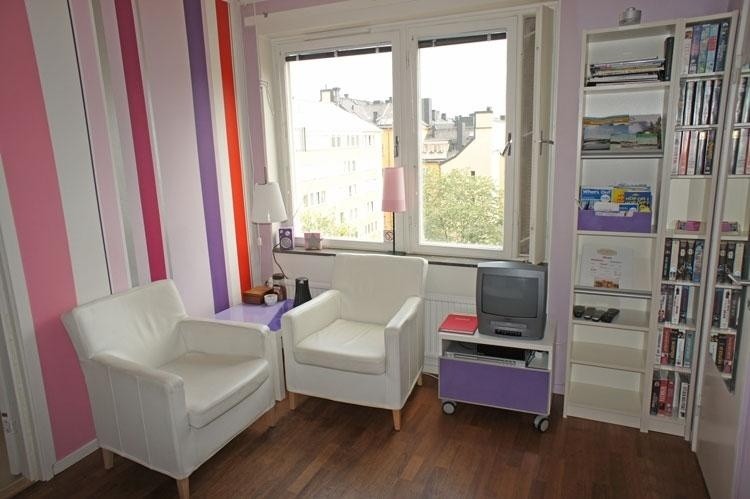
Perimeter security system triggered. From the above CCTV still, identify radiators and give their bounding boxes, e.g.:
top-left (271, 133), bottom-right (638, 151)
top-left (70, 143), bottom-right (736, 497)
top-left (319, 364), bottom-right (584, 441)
top-left (267, 278), bottom-right (476, 376)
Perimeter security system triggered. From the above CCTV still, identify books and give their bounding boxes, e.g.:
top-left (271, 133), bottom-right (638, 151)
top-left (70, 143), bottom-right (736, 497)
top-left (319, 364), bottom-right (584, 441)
top-left (648, 219), bottom-right (750, 418)
top-left (576, 17), bottom-right (749, 216)
top-left (438, 314), bottom-right (478, 335)
top-left (578, 242), bottom-right (637, 293)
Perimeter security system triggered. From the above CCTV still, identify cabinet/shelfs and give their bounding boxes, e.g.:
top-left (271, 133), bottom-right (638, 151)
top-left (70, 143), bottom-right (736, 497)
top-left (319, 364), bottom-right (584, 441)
top-left (437, 319), bottom-right (557, 433)
top-left (561, 9), bottom-right (739, 442)
top-left (708, 69), bottom-right (750, 380)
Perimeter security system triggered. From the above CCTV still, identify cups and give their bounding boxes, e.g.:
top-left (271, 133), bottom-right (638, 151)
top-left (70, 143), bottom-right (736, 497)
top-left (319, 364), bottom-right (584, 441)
top-left (264, 294), bottom-right (278, 306)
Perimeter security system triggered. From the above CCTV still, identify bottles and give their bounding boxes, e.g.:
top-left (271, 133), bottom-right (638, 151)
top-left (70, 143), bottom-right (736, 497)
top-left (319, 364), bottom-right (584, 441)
top-left (273, 272), bottom-right (287, 301)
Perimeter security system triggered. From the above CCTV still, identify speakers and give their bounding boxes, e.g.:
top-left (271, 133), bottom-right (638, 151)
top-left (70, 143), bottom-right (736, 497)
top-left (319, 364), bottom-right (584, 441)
top-left (279, 228), bottom-right (294, 251)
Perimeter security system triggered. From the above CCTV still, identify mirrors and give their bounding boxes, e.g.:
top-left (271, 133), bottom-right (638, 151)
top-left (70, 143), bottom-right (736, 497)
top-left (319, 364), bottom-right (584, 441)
top-left (708, 1), bottom-right (750, 394)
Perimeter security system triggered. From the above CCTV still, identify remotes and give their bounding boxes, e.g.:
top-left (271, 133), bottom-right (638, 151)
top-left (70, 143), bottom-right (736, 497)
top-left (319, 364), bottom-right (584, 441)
top-left (584, 307), bottom-right (596, 319)
top-left (573, 305), bottom-right (585, 318)
top-left (592, 309), bottom-right (605, 322)
top-left (600, 308), bottom-right (620, 323)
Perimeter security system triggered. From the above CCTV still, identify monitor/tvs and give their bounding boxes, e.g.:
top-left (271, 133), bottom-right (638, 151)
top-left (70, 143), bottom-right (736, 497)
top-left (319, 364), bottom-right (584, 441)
top-left (476, 260), bottom-right (547, 341)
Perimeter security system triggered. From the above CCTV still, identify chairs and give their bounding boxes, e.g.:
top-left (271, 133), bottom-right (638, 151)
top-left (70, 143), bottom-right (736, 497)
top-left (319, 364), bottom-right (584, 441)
top-left (280, 252), bottom-right (429, 431)
top-left (61, 278), bottom-right (276, 499)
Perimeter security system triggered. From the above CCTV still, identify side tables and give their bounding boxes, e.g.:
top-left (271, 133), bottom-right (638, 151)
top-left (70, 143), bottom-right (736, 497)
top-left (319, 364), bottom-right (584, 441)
top-left (214, 299), bottom-right (300, 402)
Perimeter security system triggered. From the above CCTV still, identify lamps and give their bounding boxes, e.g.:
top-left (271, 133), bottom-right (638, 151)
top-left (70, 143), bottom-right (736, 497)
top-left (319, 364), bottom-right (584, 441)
top-left (252, 166), bottom-right (288, 224)
top-left (380, 166), bottom-right (407, 257)
